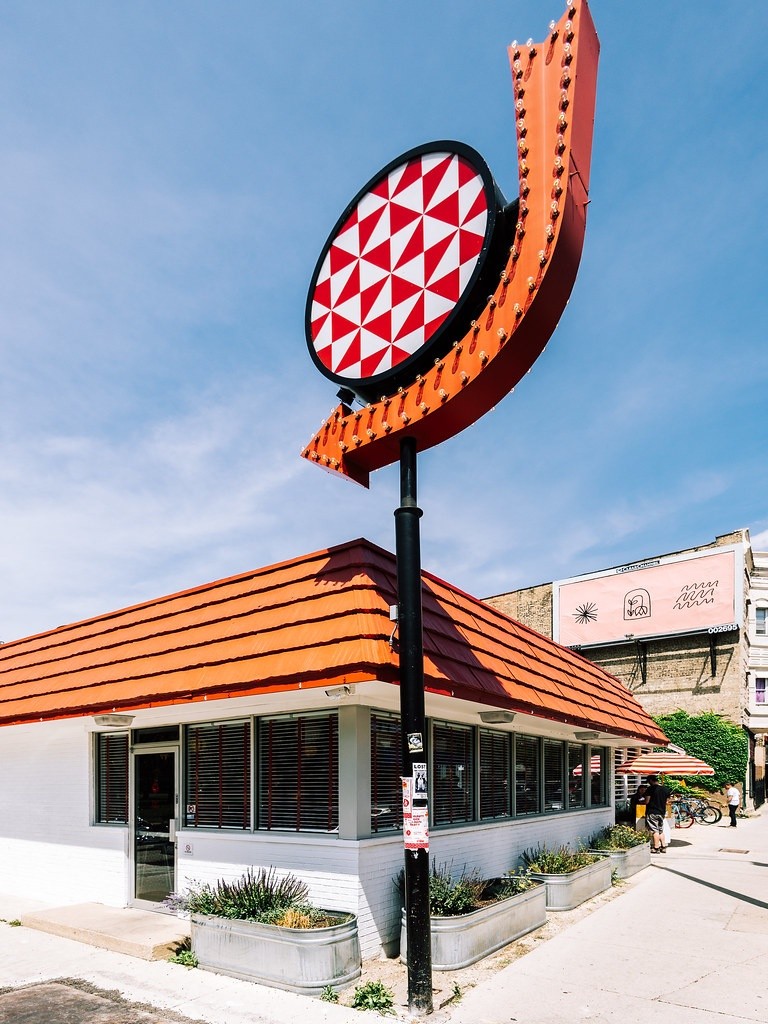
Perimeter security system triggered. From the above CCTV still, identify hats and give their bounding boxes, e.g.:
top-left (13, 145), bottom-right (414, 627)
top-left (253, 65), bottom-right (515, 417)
top-left (645, 775), bottom-right (656, 783)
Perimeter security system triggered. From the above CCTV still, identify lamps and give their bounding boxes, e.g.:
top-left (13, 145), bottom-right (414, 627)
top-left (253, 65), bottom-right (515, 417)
top-left (478, 710), bottom-right (517, 723)
top-left (92, 713), bottom-right (136, 727)
top-left (574, 730), bottom-right (600, 740)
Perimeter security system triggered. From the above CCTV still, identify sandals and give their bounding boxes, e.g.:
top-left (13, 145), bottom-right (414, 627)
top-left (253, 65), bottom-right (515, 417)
top-left (650, 847), bottom-right (660, 854)
top-left (659, 845), bottom-right (666, 853)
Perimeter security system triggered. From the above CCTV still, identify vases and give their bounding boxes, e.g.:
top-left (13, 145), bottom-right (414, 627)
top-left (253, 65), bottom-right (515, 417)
top-left (190, 841), bottom-right (652, 988)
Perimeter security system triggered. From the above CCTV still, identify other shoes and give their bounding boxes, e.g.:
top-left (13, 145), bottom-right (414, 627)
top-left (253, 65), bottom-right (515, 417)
top-left (730, 822), bottom-right (737, 827)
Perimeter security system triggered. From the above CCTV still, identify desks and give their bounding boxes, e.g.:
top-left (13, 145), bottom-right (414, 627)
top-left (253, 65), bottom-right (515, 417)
top-left (135, 828), bottom-right (169, 840)
top-left (635, 804), bottom-right (673, 847)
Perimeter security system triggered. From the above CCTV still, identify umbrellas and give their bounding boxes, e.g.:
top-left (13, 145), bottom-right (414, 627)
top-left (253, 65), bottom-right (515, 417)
top-left (572, 754), bottom-right (603, 778)
top-left (616, 751), bottom-right (715, 777)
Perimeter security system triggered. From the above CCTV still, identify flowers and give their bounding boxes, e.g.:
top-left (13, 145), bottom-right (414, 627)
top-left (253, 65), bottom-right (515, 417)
top-left (186, 822), bottom-right (652, 933)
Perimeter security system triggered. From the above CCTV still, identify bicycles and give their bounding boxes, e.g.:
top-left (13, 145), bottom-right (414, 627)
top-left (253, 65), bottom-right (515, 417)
top-left (669, 793), bottom-right (722, 829)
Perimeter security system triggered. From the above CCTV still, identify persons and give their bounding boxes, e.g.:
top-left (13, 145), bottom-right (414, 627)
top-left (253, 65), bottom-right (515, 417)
top-left (643, 775), bottom-right (668, 854)
top-left (629, 785), bottom-right (644, 826)
top-left (724, 781), bottom-right (741, 827)
top-left (672, 790), bottom-right (688, 821)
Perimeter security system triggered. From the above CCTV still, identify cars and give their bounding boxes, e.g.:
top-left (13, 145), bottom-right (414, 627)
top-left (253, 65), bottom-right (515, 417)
top-left (510, 767), bottom-right (590, 814)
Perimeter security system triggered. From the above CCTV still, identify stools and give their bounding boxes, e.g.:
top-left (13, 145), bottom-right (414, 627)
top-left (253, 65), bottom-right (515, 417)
top-left (140, 843), bottom-right (174, 893)
